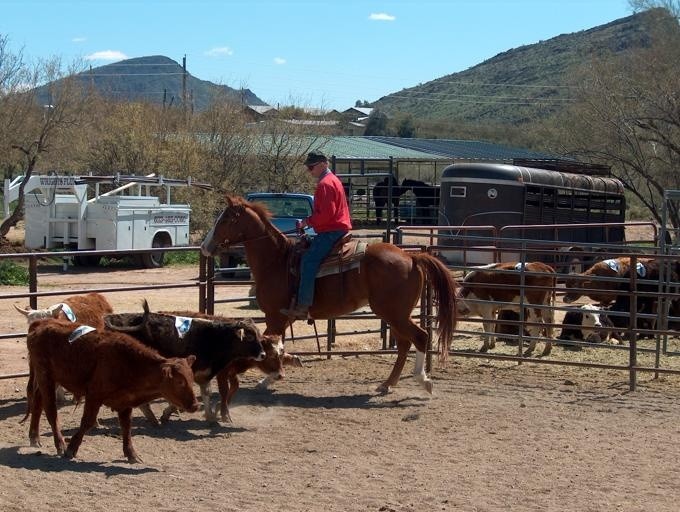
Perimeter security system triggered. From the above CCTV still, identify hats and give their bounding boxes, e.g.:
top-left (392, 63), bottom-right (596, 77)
top-left (303, 148), bottom-right (328, 167)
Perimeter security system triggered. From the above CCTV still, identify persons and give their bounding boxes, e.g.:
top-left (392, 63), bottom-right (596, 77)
top-left (279, 150), bottom-right (351, 321)
top-left (391, 177), bottom-right (401, 224)
top-left (373, 177), bottom-right (387, 226)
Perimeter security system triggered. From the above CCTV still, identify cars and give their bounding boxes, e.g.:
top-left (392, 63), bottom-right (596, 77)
top-left (221, 192), bottom-right (316, 277)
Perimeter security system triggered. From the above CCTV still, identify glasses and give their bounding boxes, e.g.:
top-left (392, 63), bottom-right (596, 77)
top-left (308, 163), bottom-right (321, 171)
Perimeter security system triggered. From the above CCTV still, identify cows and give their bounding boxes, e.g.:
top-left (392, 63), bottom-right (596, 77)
top-left (155, 310), bottom-right (286, 423)
top-left (558, 257), bottom-right (679, 351)
top-left (18, 319), bottom-right (202, 464)
top-left (494, 305), bottom-right (532, 342)
top-left (453, 261), bottom-right (556, 357)
top-left (13, 293), bottom-right (114, 404)
top-left (103, 299), bottom-right (267, 429)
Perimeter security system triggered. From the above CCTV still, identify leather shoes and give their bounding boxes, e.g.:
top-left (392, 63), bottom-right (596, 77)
top-left (278, 302), bottom-right (310, 321)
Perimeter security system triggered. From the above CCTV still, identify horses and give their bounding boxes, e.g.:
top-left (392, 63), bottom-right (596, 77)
top-left (400, 177), bottom-right (440, 223)
top-left (372, 176), bottom-right (401, 223)
top-left (200, 195), bottom-right (457, 396)
